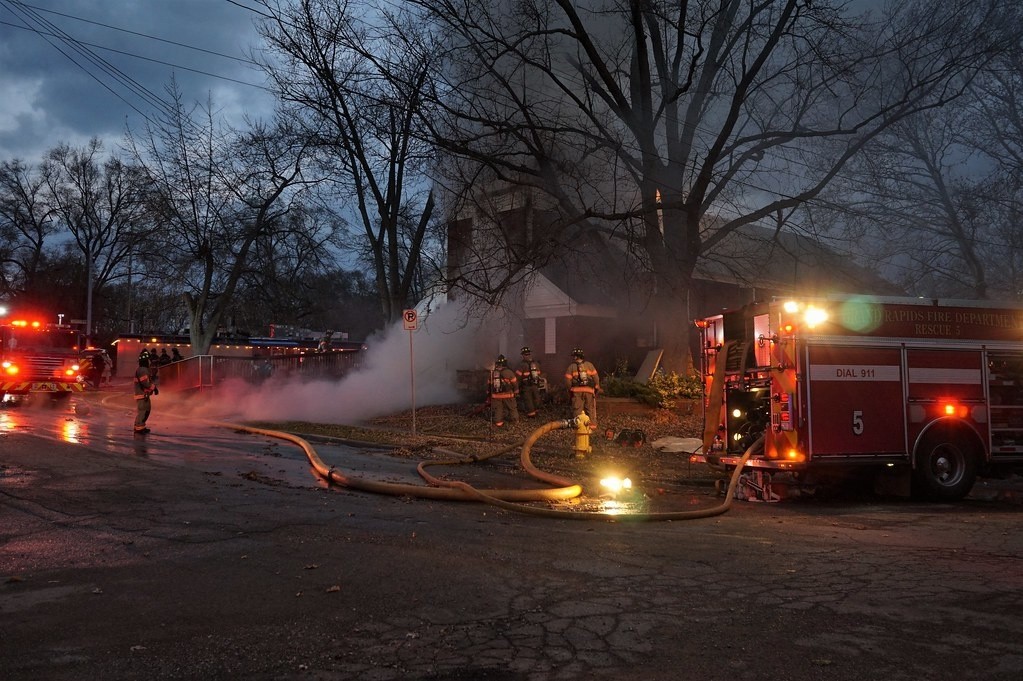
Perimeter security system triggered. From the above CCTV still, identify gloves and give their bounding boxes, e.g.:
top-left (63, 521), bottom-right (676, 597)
top-left (149, 388), bottom-right (158, 395)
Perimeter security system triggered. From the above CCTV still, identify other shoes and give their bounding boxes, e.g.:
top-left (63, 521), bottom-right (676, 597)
top-left (134, 428), bottom-right (150, 436)
top-left (527, 412), bottom-right (539, 417)
top-left (588, 423), bottom-right (598, 430)
top-left (496, 422), bottom-right (505, 427)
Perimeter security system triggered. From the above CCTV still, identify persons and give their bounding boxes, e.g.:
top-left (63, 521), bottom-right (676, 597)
top-left (564, 345), bottom-right (604, 427)
top-left (513, 346), bottom-right (542, 416)
top-left (140, 347), bottom-right (184, 384)
top-left (487, 354), bottom-right (520, 426)
top-left (92, 348), bottom-right (105, 388)
top-left (318, 328), bottom-right (336, 372)
top-left (134, 352), bottom-right (158, 434)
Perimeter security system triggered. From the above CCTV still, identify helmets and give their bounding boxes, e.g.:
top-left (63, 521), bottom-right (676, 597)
top-left (138, 352), bottom-right (151, 360)
top-left (496, 354), bottom-right (506, 365)
top-left (568, 348), bottom-right (584, 356)
top-left (326, 329), bottom-right (333, 334)
top-left (520, 347), bottom-right (531, 354)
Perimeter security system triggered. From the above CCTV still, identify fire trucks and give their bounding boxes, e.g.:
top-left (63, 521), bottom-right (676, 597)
top-left (1, 315), bottom-right (85, 404)
top-left (696, 284), bottom-right (1021, 492)
